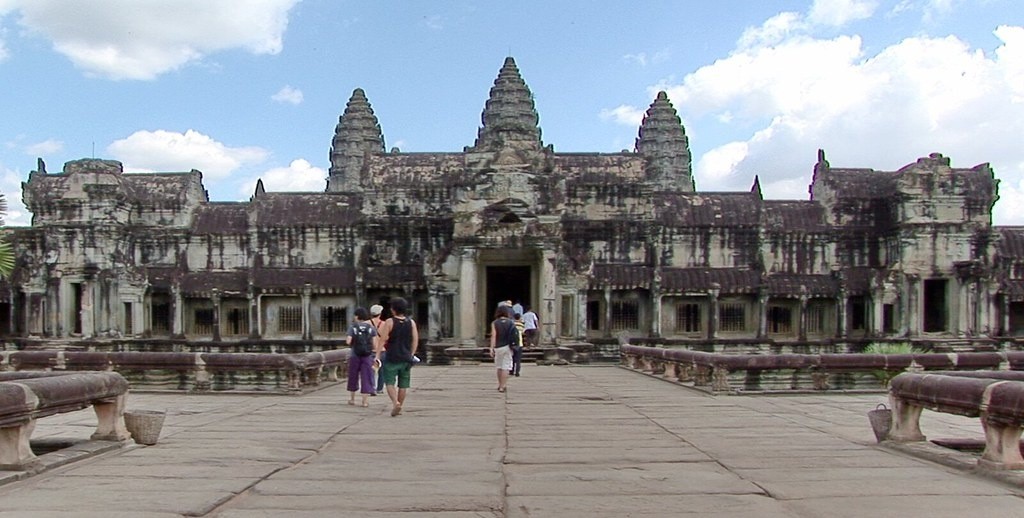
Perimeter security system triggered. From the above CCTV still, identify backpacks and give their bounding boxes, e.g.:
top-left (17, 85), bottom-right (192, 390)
top-left (353, 324), bottom-right (372, 356)
top-left (508, 319), bottom-right (520, 349)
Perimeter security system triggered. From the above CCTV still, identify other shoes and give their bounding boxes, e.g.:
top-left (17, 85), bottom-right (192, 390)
top-left (348, 400), bottom-right (354, 405)
top-left (362, 402), bottom-right (369, 407)
top-left (391, 406), bottom-right (400, 416)
top-left (498, 387), bottom-right (506, 392)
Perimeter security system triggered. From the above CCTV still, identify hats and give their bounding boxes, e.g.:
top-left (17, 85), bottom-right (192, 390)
top-left (369, 304), bottom-right (383, 315)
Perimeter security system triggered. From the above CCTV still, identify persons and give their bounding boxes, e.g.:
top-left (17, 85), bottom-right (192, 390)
top-left (373, 297), bottom-right (419, 416)
top-left (345, 304), bottom-right (389, 406)
top-left (489, 300), bottom-right (540, 392)
